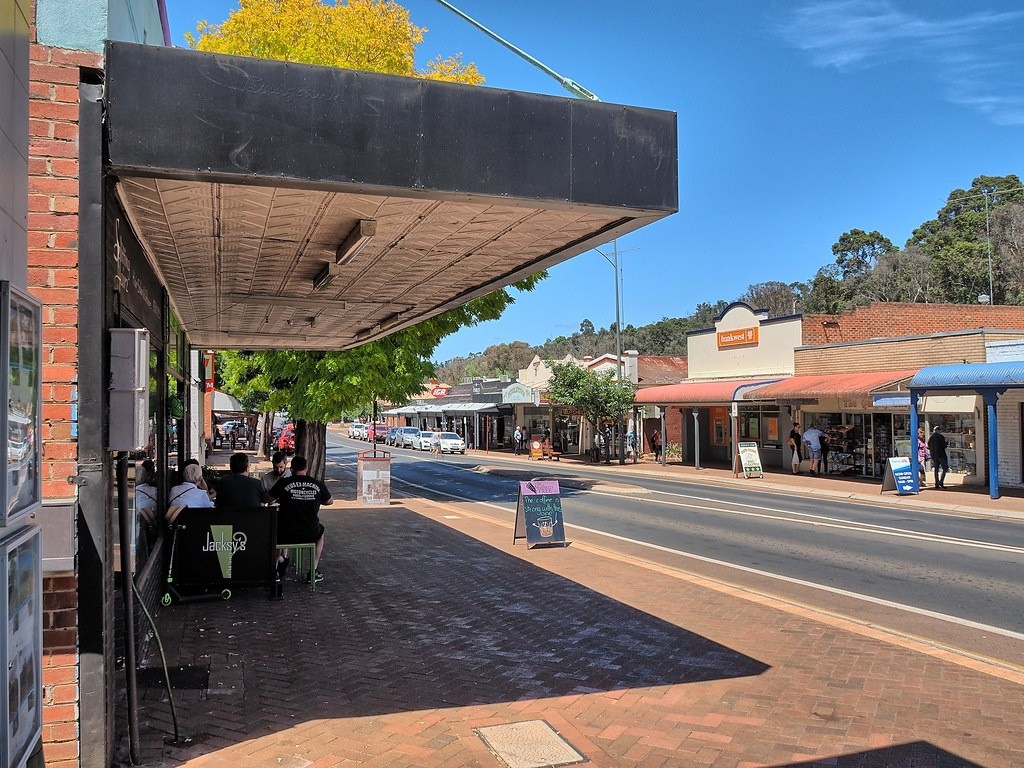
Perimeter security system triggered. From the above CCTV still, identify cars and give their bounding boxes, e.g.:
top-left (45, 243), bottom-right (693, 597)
top-left (221, 421), bottom-right (247, 437)
top-left (359, 425), bottom-right (370, 441)
top-left (283, 434), bottom-right (295, 456)
top-left (276, 429), bottom-right (294, 452)
top-left (347, 424), bottom-right (364, 439)
top-left (271, 411), bottom-right (297, 451)
top-left (430, 432), bottom-right (466, 455)
top-left (394, 427), bottom-right (420, 449)
top-left (410, 431), bottom-right (435, 452)
top-left (367, 425), bottom-right (388, 443)
top-left (385, 428), bottom-right (399, 446)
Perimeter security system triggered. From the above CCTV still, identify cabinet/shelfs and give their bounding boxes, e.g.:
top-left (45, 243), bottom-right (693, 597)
top-left (929, 432), bottom-right (976, 476)
top-left (819, 424), bottom-right (855, 476)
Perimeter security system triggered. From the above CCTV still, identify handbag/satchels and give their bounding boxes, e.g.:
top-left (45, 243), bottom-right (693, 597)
top-left (787, 432), bottom-right (795, 445)
top-left (792, 448), bottom-right (800, 465)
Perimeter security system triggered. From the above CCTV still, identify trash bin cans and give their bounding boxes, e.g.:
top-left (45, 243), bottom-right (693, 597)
top-left (357, 449), bottom-right (392, 505)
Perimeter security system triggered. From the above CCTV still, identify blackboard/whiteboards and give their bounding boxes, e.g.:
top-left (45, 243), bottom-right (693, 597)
top-left (513, 480), bottom-right (566, 542)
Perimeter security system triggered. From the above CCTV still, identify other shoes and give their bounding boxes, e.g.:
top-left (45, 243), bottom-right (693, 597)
top-left (920, 480), bottom-right (929, 487)
top-left (934, 481), bottom-right (947, 489)
top-left (794, 470), bottom-right (803, 475)
top-left (809, 470), bottom-right (817, 477)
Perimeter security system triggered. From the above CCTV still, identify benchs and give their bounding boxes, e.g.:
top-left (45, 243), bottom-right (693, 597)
top-left (169, 506), bottom-right (277, 598)
top-left (528, 444), bottom-right (562, 461)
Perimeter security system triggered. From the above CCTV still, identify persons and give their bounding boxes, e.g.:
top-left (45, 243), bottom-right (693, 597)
top-left (801, 425), bottom-right (830, 475)
top-left (542, 426), bottom-right (550, 442)
top-left (168, 458), bottom-right (217, 508)
top-left (790, 422), bottom-right (803, 475)
top-left (513, 425), bottom-right (521, 455)
top-left (651, 429), bottom-right (662, 463)
top-left (214, 453), bottom-right (266, 552)
top-left (625, 426), bottom-right (644, 459)
top-left (261, 452), bottom-right (333, 583)
top-left (918, 427), bottom-right (927, 487)
top-left (522, 426), bottom-right (529, 451)
top-left (209, 423), bottom-right (261, 452)
top-left (928, 426), bottom-right (949, 489)
top-left (817, 429), bottom-right (830, 475)
top-left (136, 459), bottom-right (157, 509)
top-left (430, 427), bottom-right (442, 458)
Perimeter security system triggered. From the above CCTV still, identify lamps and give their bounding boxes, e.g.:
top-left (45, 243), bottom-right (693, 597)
top-left (228, 331), bottom-right (308, 341)
top-left (978, 294), bottom-right (990, 305)
top-left (336, 219), bottom-right (376, 266)
top-left (313, 262), bottom-right (342, 292)
top-left (228, 294), bottom-right (348, 310)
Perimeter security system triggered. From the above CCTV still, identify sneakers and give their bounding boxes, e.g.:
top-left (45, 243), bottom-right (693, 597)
top-left (306, 570), bottom-right (324, 584)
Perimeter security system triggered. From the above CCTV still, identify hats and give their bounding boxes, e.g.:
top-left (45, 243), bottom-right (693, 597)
top-left (165, 502), bottom-right (187, 524)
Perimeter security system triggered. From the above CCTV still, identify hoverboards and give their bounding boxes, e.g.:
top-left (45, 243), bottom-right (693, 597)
top-left (160, 524), bottom-right (232, 606)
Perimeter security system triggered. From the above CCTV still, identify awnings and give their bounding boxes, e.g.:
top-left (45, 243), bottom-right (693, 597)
top-left (381, 403), bottom-right (512, 454)
top-left (922, 396), bottom-right (977, 413)
top-left (211, 390), bottom-right (245, 411)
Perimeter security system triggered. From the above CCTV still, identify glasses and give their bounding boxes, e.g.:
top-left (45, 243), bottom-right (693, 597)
top-left (796, 426), bottom-right (800, 428)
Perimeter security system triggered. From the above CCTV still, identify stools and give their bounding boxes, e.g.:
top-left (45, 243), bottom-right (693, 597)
top-left (275, 542), bottom-right (316, 592)
